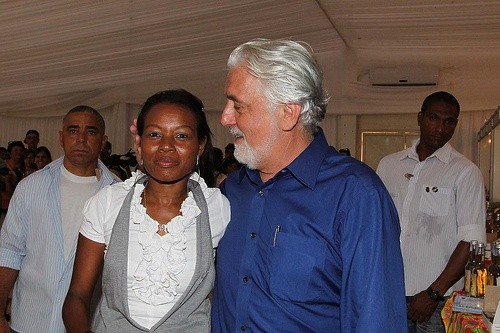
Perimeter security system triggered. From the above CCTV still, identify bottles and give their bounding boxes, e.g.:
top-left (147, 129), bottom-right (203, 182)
top-left (464, 240), bottom-right (478, 296)
top-left (470, 243), bottom-right (487, 298)
top-left (486, 197), bottom-right (500, 244)
top-left (488, 243), bottom-right (500, 286)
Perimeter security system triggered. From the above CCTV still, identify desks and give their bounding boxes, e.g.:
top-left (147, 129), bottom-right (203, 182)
top-left (441, 290), bottom-right (490, 333)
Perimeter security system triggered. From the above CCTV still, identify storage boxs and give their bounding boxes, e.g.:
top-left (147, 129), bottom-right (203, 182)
top-left (480, 285), bottom-right (500, 333)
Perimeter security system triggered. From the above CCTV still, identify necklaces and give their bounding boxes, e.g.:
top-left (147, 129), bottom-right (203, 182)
top-left (143, 181), bottom-right (188, 231)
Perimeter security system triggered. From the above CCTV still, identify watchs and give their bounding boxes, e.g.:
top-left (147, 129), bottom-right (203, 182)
top-left (427, 286), bottom-right (445, 303)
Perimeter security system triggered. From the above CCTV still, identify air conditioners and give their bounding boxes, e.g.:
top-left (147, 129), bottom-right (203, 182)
top-left (367, 66), bottom-right (438, 90)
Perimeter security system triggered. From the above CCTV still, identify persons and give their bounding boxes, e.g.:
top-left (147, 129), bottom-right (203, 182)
top-left (0, 106), bottom-right (124, 333)
top-left (100, 142), bottom-right (138, 182)
top-left (0, 130), bottom-right (53, 227)
top-left (211, 143), bottom-right (241, 188)
top-left (130, 38), bottom-right (409, 333)
top-left (375, 91), bottom-right (487, 333)
top-left (62, 90), bottom-right (231, 333)
